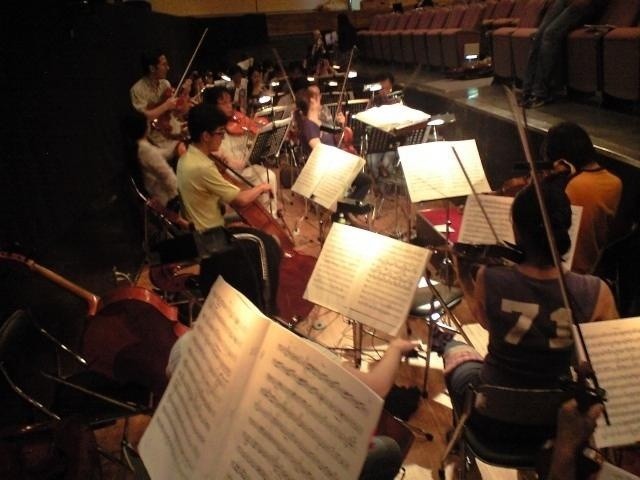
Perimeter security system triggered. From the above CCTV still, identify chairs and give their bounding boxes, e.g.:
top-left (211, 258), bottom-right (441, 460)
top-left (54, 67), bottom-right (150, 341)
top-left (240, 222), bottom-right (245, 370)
top-left (0, 170), bottom-right (640, 480)
top-left (359, 1), bottom-right (639, 108)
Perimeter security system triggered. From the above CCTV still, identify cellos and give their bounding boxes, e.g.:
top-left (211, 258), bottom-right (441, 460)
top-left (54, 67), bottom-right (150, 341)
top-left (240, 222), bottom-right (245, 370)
top-left (0, 251), bottom-right (190, 408)
top-left (151, 119), bottom-right (296, 253)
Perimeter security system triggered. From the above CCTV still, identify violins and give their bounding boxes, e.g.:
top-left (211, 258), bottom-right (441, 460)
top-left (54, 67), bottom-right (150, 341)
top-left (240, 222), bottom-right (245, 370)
top-left (424, 239), bottom-right (523, 266)
top-left (224, 111), bottom-right (269, 135)
top-left (478, 169), bottom-right (553, 198)
top-left (334, 110), bottom-right (358, 155)
top-left (533, 362), bottom-right (605, 479)
top-left (149, 71), bottom-right (198, 136)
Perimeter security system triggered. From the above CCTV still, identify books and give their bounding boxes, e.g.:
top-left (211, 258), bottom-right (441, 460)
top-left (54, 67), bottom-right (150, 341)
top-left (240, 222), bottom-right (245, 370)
top-left (355, 103), bottom-right (432, 132)
top-left (138, 274), bottom-right (385, 480)
top-left (302, 224), bottom-right (434, 338)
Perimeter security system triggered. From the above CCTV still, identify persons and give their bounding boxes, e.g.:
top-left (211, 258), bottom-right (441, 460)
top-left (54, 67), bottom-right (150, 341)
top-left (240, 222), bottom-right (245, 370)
top-left (443, 122), bottom-right (623, 480)
top-left (517, 1), bottom-right (604, 108)
top-left (128, 29), bottom-right (406, 235)
top-left (167, 324), bottom-right (421, 480)
